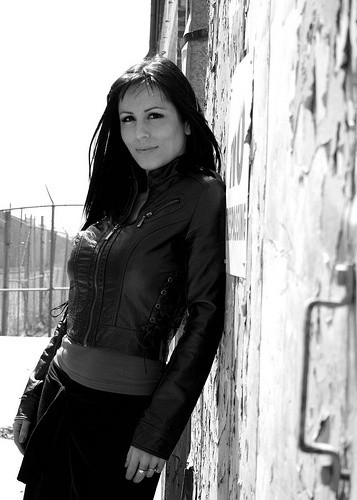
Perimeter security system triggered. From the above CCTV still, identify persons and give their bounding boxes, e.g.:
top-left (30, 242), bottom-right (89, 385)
top-left (13, 57), bottom-right (226, 500)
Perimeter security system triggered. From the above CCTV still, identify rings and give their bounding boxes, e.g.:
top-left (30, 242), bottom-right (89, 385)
top-left (138, 468), bottom-right (147, 474)
top-left (148, 468), bottom-right (156, 472)
